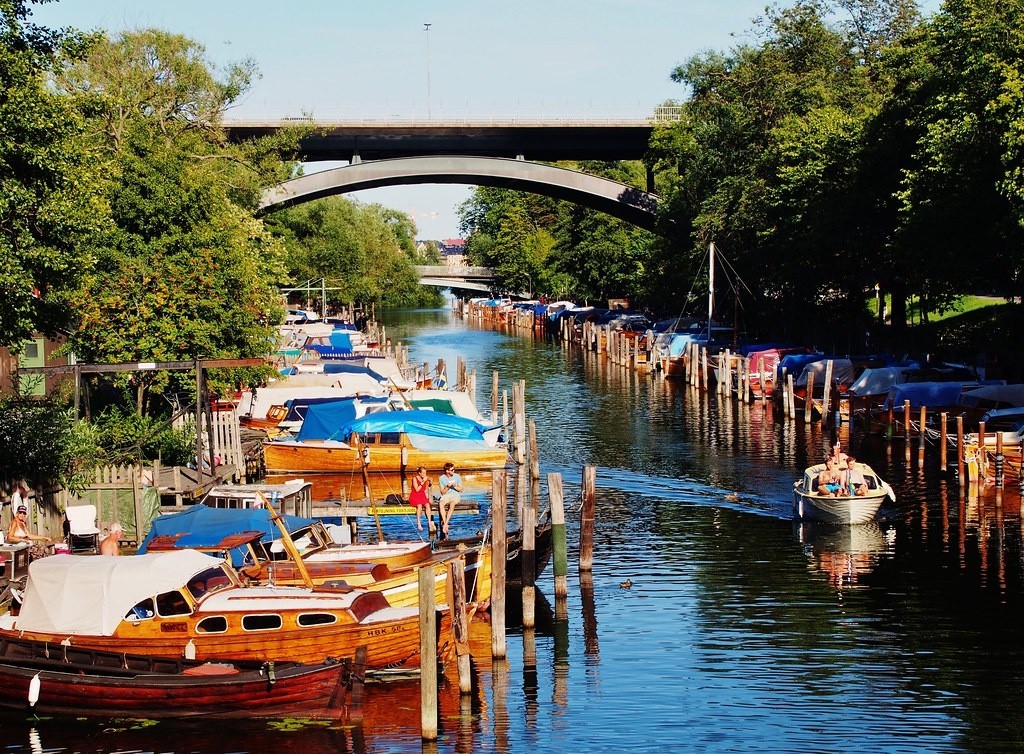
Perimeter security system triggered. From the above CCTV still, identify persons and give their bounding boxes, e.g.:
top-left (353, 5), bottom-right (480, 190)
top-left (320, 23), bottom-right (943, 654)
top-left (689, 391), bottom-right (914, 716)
top-left (439, 463), bottom-right (463, 534)
top-left (7, 506), bottom-right (51, 562)
top-left (818, 457), bottom-right (842, 497)
top-left (409, 466), bottom-right (433, 530)
top-left (11, 476), bottom-right (30, 529)
top-left (840, 457), bottom-right (868, 497)
top-left (99, 523), bottom-right (123, 556)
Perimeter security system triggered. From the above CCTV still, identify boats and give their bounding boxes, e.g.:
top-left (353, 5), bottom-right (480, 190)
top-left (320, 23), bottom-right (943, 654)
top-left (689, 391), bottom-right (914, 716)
top-left (265, 470), bottom-right (509, 502)
top-left (0, 549), bottom-right (479, 670)
top-left (135, 431), bottom-right (553, 608)
top-left (470, 242), bottom-right (1024, 515)
top-left (0, 637), bottom-right (352, 720)
top-left (813, 523), bottom-right (889, 589)
top-left (206, 277), bottom-right (513, 471)
top-left (793, 436), bottom-right (887, 525)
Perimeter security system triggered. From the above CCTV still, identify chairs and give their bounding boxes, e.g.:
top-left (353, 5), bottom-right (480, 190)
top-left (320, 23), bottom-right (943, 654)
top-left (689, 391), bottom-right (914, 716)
top-left (66, 505), bottom-right (100, 555)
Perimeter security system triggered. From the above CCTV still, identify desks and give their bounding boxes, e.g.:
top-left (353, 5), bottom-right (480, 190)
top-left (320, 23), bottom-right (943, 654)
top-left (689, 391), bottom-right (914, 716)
top-left (0, 546), bottom-right (30, 580)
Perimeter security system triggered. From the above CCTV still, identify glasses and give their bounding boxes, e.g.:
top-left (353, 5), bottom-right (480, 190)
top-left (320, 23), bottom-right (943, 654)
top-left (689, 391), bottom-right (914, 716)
top-left (19, 512), bottom-right (26, 515)
top-left (448, 469), bottom-right (455, 472)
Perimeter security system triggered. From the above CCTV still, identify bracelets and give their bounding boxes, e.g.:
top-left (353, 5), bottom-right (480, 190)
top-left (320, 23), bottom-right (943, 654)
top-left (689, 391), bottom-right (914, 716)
top-left (420, 484), bottom-right (423, 486)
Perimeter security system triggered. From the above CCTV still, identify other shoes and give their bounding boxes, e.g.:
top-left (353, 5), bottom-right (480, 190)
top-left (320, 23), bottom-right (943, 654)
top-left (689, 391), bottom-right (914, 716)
top-left (442, 524), bottom-right (449, 533)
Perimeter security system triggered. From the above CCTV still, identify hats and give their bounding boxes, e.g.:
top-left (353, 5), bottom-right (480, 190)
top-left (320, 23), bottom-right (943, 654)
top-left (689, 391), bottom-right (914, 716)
top-left (17, 506), bottom-right (26, 513)
top-left (19, 481), bottom-right (28, 490)
